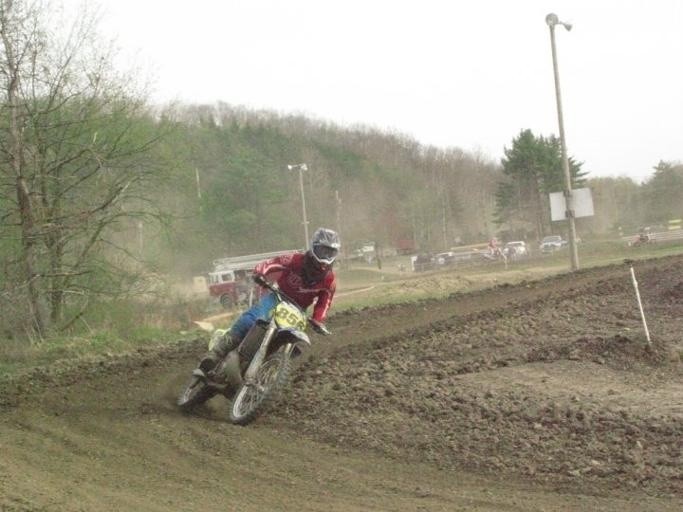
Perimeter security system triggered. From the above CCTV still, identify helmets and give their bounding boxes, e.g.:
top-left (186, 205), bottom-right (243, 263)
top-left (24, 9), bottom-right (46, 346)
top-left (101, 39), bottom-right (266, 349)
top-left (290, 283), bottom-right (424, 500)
top-left (310, 227), bottom-right (341, 272)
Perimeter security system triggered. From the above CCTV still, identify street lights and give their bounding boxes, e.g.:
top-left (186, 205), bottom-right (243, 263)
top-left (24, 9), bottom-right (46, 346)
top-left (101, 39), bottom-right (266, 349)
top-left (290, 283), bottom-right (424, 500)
top-left (543, 12), bottom-right (579, 272)
top-left (286, 162), bottom-right (311, 250)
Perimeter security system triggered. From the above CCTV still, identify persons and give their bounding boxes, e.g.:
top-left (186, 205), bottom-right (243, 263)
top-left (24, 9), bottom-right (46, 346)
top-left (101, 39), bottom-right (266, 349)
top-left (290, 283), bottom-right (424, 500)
top-left (506, 243), bottom-right (517, 262)
top-left (487, 236), bottom-right (495, 255)
top-left (193, 225), bottom-right (340, 380)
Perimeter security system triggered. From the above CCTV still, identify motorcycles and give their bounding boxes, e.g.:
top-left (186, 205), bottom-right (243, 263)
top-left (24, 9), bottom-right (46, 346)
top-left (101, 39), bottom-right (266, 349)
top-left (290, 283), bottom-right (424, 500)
top-left (174, 270), bottom-right (334, 428)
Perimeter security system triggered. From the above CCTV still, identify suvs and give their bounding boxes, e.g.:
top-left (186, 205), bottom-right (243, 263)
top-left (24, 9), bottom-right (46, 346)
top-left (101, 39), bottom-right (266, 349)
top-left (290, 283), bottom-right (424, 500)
top-left (539, 235), bottom-right (562, 254)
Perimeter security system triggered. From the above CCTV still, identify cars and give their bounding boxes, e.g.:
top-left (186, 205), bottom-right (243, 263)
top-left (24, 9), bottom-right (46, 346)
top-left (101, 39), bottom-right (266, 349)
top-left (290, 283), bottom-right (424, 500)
top-left (501, 239), bottom-right (526, 256)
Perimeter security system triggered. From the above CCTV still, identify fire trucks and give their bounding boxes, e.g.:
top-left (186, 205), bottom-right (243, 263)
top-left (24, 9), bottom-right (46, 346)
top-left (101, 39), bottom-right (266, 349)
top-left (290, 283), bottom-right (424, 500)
top-left (205, 248), bottom-right (302, 308)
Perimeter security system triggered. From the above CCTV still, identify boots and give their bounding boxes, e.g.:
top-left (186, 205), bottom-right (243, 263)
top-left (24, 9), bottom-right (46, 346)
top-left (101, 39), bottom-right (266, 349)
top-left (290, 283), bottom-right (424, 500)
top-left (199, 329), bottom-right (239, 373)
